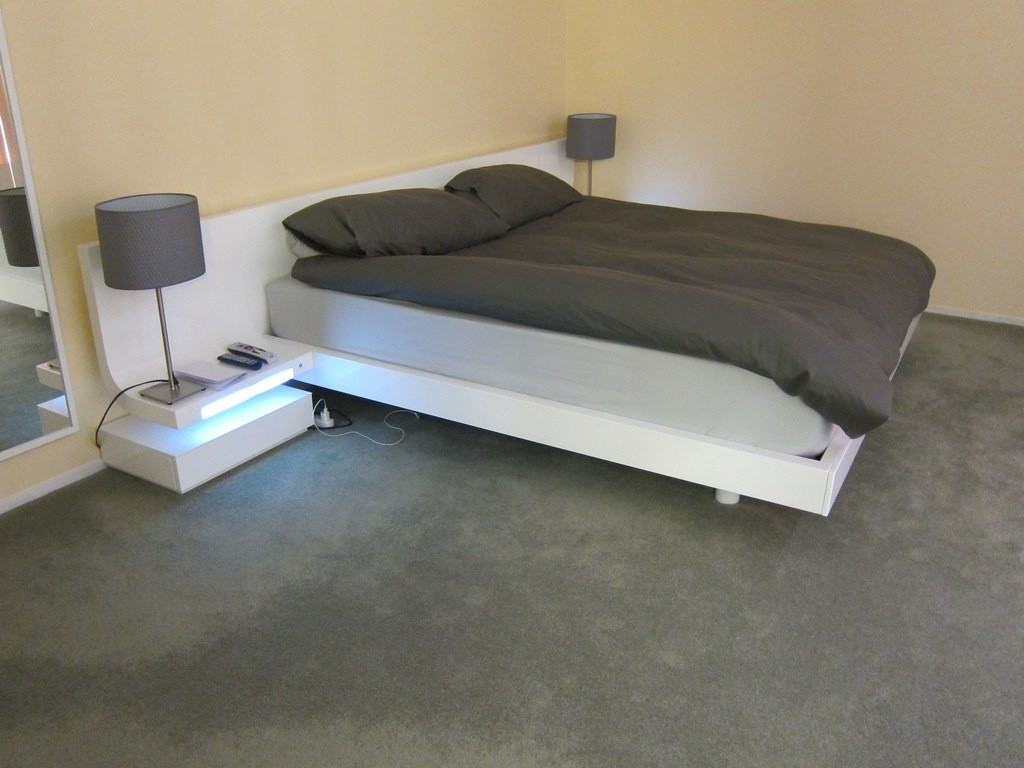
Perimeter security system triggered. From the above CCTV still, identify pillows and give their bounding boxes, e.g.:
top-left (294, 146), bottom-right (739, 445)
top-left (280, 162), bottom-right (588, 256)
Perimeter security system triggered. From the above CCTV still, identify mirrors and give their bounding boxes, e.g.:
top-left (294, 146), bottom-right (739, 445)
top-left (0, 4), bottom-right (82, 464)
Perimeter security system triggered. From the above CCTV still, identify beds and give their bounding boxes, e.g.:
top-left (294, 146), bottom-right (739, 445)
top-left (73, 138), bottom-right (939, 519)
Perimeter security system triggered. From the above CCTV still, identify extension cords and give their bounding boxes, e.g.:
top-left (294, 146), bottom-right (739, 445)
top-left (312, 414), bottom-right (335, 428)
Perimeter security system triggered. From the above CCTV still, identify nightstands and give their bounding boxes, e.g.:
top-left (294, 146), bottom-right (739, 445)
top-left (95, 333), bottom-right (319, 497)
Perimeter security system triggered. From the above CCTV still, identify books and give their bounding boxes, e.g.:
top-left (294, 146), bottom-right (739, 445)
top-left (172, 363), bottom-right (248, 391)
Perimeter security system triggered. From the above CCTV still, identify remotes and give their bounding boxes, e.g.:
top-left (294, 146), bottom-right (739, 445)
top-left (227, 341), bottom-right (280, 363)
top-left (217, 353), bottom-right (263, 370)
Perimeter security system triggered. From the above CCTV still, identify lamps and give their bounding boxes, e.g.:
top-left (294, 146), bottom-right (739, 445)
top-left (92, 189), bottom-right (209, 404)
top-left (565, 111), bottom-right (619, 196)
top-left (1, 187), bottom-right (38, 268)
